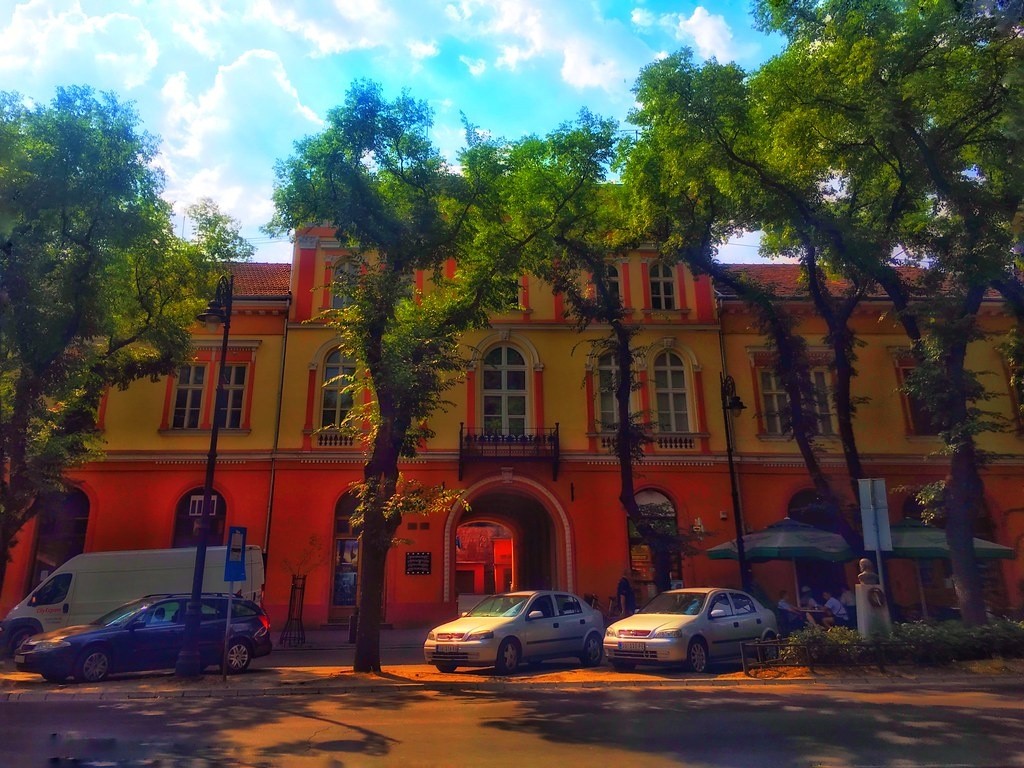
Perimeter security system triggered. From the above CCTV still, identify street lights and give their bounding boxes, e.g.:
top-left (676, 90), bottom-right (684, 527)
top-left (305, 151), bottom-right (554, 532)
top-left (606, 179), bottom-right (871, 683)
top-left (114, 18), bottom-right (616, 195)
top-left (167, 274), bottom-right (236, 678)
top-left (718, 369), bottom-right (755, 598)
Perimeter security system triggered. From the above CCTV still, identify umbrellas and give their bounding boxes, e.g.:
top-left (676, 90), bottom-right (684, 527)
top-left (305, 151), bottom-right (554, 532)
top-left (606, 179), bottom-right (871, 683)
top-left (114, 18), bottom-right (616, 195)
top-left (843, 516), bottom-right (1015, 622)
top-left (705, 517), bottom-right (855, 625)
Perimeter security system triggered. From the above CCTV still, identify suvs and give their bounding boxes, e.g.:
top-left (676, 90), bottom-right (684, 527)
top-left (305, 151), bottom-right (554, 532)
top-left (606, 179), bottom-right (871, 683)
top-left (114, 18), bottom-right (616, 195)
top-left (11, 591), bottom-right (275, 684)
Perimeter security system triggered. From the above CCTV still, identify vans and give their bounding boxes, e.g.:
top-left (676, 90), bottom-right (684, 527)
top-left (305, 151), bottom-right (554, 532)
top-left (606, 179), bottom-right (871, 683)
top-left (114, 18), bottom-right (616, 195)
top-left (0, 544), bottom-right (267, 661)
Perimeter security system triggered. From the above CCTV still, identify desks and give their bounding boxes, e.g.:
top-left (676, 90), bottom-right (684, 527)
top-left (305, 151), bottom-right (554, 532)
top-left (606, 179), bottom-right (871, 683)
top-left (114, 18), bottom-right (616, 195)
top-left (800, 609), bottom-right (824, 619)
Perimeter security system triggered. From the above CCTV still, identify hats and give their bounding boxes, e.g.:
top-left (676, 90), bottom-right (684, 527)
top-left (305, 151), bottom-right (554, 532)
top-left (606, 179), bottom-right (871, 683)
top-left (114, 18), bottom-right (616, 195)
top-left (802, 586), bottom-right (812, 593)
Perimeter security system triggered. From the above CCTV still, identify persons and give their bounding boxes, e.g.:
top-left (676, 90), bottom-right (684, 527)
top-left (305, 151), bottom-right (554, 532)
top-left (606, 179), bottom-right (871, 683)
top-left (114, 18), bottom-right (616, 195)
top-left (616, 568), bottom-right (635, 618)
top-left (768, 586), bottom-right (857, 633)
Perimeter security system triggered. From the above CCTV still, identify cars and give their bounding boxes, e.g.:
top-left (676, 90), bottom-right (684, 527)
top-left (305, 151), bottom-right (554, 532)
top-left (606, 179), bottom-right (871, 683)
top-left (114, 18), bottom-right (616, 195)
top-left (601, 586), bottom-right (783, 675)
top-left (422, 591), bottom-right (603, 676)
